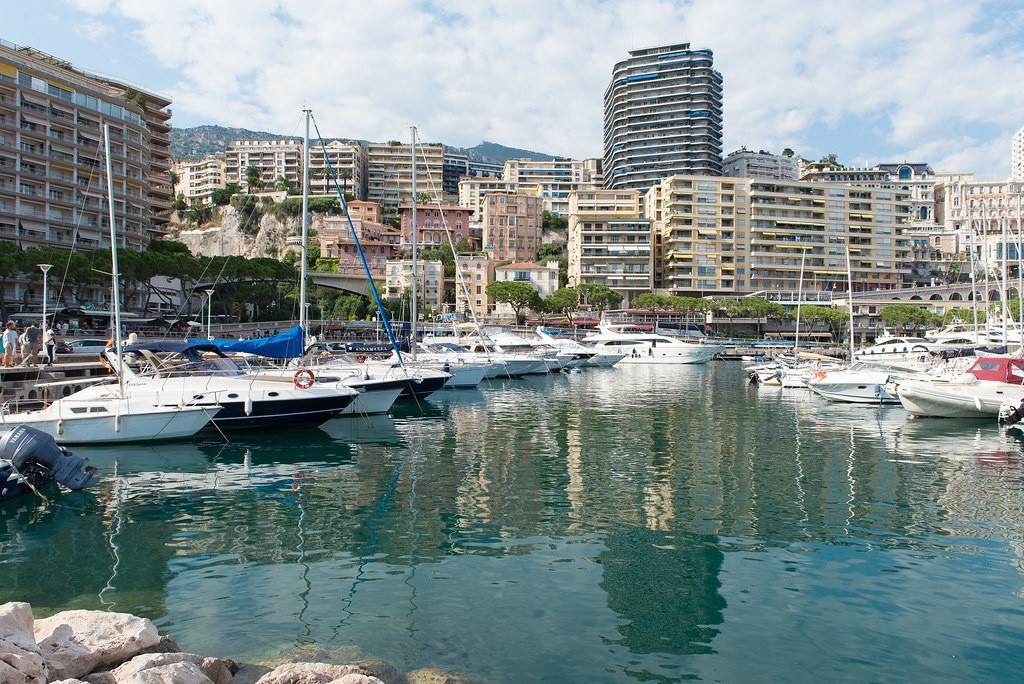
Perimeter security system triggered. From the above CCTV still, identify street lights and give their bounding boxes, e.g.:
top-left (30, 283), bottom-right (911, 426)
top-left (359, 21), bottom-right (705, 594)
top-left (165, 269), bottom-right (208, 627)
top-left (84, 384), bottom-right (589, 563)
top-left (304, 302), bottom-right (311, 345)
top-left (374, 310), bottom-right (397, 344)
top-left (36, 263), bottom-right (53, 356)
top-left (206, 289), bottom-right (215, 341)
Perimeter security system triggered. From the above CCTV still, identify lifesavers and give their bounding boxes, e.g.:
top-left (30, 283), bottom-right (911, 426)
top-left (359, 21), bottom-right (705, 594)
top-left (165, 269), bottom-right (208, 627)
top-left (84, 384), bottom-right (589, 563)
top-left (293, 369), bottom-right (316, 390)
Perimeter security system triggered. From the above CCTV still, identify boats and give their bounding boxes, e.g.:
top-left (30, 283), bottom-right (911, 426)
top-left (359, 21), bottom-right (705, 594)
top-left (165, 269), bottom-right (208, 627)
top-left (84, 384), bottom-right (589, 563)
top-left (1, 125), bottom-right (226, 446)
top-left (583, 320), bottom-right (725, 366)
top-left (104, 107), bottom-right (628, 433)
top-left (739, 187), bottom-right (1023, 447)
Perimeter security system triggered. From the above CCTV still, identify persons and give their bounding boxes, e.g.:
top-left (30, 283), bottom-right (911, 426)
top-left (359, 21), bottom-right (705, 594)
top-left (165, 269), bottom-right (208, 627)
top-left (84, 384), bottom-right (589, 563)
top-left (0, 319), bottom-right (73, 367)
top-left (811, 357), bottom-right (824, 371)
top-left (175, 324), bottom-right (192, 339)
top-left (308, 334), bottom-right (318, 345)
top-left (73, 319), bottom-right (141, 341)
top-left (221, 327), bottom-right (280, 341)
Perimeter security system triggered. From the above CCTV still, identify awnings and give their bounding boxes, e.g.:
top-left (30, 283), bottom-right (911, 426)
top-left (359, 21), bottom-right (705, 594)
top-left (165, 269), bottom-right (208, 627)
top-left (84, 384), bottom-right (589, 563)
top-left (11, 310), bottom-right (54, 318)
top-left (80, 309), bottom-right (139, 318)
top-left (120, 318), bottom-right (157, 324)
top-left (165, 320), bottom-right (204, 327)
